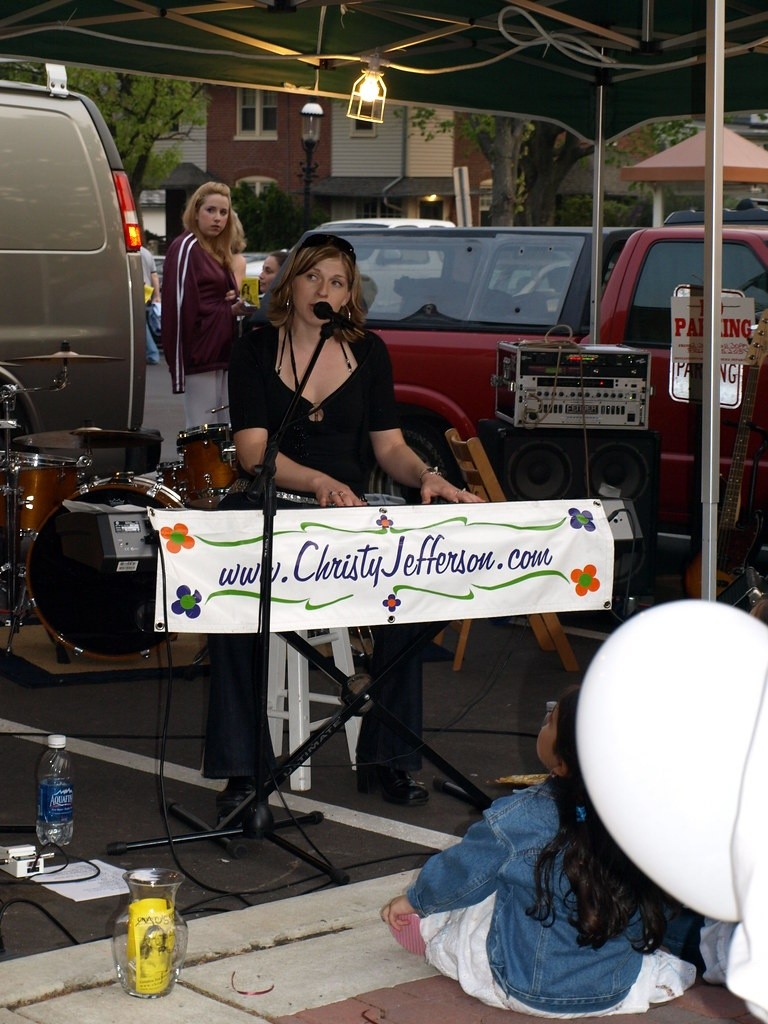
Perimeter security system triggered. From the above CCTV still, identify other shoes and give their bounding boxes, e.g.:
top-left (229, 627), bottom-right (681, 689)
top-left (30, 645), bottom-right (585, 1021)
top-left (389, 914), bottom-right (427, 956)
top-left (216, 776), bottom-right (269, 808)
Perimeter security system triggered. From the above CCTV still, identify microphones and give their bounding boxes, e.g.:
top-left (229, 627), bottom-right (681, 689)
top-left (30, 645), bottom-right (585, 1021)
top-left (313, 301), bottom-right (356, 331)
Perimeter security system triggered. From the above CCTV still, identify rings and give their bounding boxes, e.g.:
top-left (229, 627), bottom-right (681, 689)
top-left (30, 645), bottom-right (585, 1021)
top-left (339, 491), bottom-right (344, 496)
top-left (329, 490), bottom-right (337, 498)
top-left (454, 487), bottom-right (466, 496)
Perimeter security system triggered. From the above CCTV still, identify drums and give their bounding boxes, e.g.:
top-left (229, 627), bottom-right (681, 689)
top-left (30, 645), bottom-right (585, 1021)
top-left (22, 473), bottom-right (187, 663)
top-left (176, 421), bottom-right (243, 508)
top-left (1, 452), bottom-right (79, 537)
top-left (156, 460), bottom-right (187, 493)
top-left (0, 368), bottom-right (41, 454)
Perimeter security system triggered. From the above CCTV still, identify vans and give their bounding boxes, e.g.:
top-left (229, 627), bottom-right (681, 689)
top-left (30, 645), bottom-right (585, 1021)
top-left (0, 59), bottom-right (164, 476)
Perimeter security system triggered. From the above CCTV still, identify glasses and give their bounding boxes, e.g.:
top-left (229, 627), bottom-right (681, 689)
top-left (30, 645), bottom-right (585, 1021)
top-left (297, 234), bottom-right (356, 265)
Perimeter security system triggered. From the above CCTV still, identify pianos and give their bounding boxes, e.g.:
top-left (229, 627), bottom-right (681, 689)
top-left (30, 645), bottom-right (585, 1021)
top-left (163, 488), bottom-right (501, 857)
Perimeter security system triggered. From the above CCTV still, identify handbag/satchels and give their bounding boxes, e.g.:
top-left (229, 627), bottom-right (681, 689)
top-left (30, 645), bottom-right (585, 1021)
top-left (148, 302), bottom-right (161, 336)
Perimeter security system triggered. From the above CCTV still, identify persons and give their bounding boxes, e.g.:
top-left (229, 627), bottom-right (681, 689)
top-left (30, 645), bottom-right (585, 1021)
top-left (160, 181), bottom-right (291, 433)
top-left (139, 925), bottom-right (170, 979)
top-left (140, 245), bottom-right (161, 365)
top-left (379, 684), bottom-right (707, 1019)
top-left (201, 232), bottom-right (487, 809)
top-left (242, 283), bottom-right (255, 305)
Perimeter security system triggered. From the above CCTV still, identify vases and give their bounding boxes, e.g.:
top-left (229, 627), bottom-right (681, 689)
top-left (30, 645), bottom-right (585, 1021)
top-left (111, 868), bottom-right (188, 999)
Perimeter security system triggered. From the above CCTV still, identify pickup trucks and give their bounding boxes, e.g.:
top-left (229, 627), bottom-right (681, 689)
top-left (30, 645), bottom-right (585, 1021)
top-left (236, 209), bottom-right (767, 574)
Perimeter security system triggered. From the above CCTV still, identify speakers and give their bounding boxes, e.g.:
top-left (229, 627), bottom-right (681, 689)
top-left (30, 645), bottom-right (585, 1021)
top-left (478, 419), bottom-right (664, 595)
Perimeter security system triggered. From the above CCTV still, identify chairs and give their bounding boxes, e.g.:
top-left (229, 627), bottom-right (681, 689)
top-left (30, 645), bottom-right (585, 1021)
top-left (267, 626), bottom-right (364, 791)
top-left (432, 427), bottom-right (582, 673)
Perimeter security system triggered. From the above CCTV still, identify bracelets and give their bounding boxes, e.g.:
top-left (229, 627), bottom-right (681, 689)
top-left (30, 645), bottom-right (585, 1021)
top-left (417, 466), bottom-right (442, 484)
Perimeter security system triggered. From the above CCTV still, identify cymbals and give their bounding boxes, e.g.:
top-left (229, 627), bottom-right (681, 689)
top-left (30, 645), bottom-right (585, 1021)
top-left (3, 350), bottom-right (127, 362)
top-left (0, 361), bottom-right (24, 367)
top-left (12, 427), bottom-right (164, 450)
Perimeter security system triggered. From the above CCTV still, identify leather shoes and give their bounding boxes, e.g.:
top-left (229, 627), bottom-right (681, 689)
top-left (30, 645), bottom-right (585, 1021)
top-left (357, 769), bottom-right (430, 807)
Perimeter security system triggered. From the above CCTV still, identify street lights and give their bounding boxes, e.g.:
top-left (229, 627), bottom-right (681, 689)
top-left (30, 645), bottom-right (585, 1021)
top-left (300, 96), bottom-right (326, 234)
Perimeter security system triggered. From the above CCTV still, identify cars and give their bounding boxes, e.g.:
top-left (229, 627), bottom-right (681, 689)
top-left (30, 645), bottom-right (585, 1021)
top-left (149, 213), bottom-right (461, 317)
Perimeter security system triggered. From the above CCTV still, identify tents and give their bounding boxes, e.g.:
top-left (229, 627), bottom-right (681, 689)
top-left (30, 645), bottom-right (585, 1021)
top-left (0, 0), bottom-right (768, 855)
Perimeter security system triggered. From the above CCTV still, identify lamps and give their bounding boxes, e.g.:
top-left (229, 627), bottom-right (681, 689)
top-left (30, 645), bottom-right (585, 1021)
top-left (346, 56), bottom-right (391, 123)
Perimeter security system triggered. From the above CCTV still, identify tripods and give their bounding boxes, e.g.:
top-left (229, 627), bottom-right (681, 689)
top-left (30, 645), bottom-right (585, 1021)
top-left (106, 321), bottom-right (350, 885)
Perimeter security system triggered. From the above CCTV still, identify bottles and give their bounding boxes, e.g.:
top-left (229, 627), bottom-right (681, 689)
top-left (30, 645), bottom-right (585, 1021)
top-left (111, 866), bottom-right (188, 999)
top-left (36, 734), bottom-right (74, 847)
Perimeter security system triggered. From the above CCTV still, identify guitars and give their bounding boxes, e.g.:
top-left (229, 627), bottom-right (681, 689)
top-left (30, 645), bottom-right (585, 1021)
top-left (682, 309), bottom-right (768, 604)
